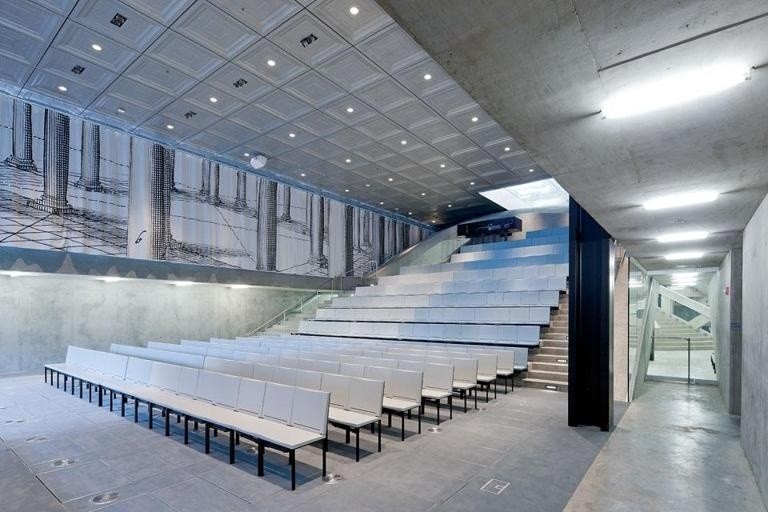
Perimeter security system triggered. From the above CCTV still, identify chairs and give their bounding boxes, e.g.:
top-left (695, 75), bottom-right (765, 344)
top-left (43, 226), bottom-right (568, 491)
